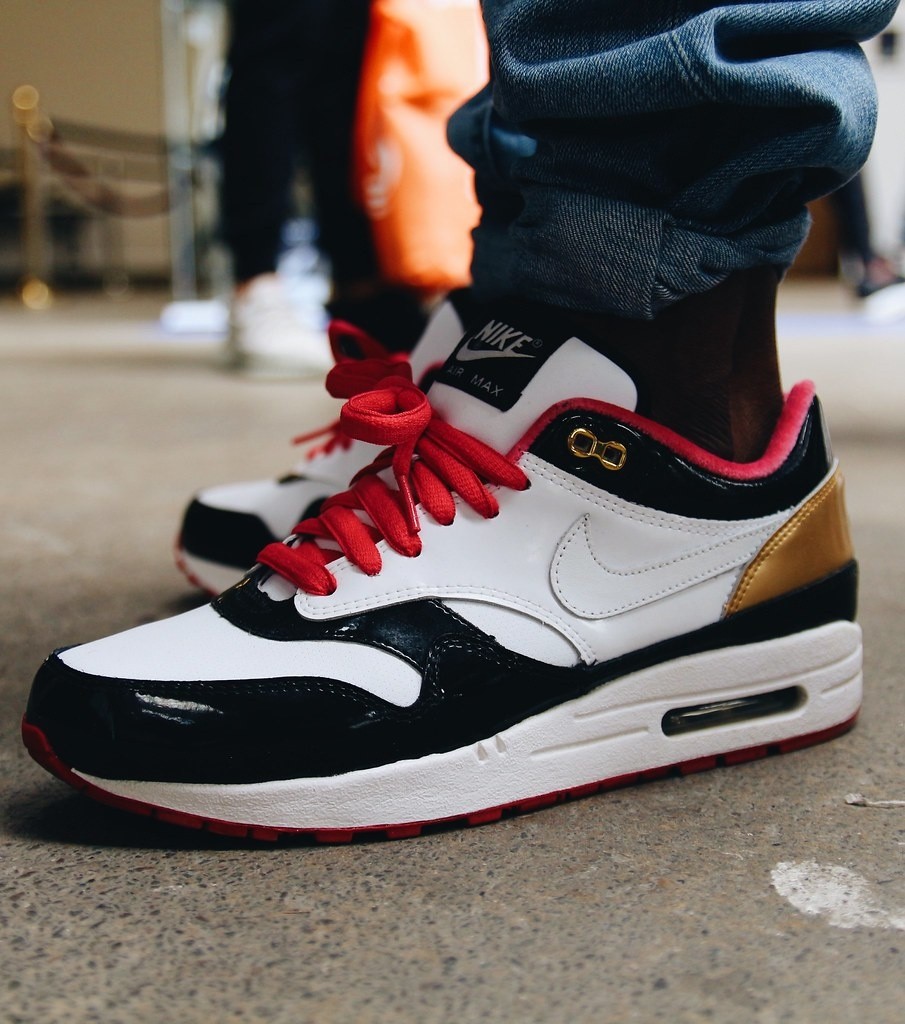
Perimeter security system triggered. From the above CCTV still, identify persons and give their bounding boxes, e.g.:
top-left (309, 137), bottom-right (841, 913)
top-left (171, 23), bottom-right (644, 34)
top-left (22, 0), bottom-right (901, 842)
top-left (161, 0), bottom-right (431, 370)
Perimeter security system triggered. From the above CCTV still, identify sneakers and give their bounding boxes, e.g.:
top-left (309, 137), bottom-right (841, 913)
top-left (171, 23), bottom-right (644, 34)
top-left (21, 282), bottom-right (863, 842)
